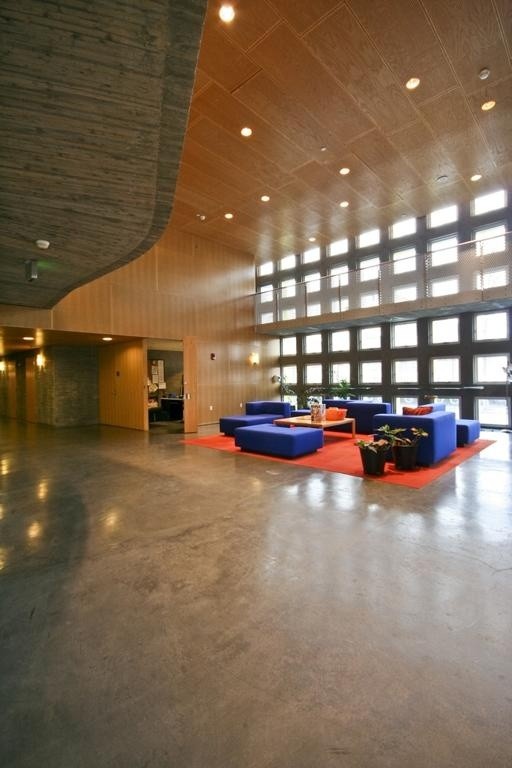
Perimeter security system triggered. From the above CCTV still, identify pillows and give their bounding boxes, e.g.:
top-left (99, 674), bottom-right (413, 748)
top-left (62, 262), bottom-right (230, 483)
top-left (403, 407), bottom-right (433, 415)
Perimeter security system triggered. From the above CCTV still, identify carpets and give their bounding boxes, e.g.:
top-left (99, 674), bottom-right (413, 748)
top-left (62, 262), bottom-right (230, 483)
top-left (178, 430), bottom-right (495, 490)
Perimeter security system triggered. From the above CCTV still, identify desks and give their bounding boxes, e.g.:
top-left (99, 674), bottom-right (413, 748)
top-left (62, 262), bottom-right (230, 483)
top-left (160, 397), bottom-right (184, 421)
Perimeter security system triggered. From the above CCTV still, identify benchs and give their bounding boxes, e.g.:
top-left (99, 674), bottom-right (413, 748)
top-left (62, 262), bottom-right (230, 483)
top-left (219, 401), bottom-right (295, 436)
top-left (234, 423), bottom-right (324, 459)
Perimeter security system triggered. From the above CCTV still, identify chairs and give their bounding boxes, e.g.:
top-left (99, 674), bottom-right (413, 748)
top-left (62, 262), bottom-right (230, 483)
top-left (322, 399), bottom-right (392, 435)
top-left (372, 411), bottom-right (457, 467)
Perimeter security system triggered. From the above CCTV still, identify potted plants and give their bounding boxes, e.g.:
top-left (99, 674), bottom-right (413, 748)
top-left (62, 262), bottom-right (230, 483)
top-left (378, 423), bottom-right (429, 471)
top-left (354, 439), bottom-right (392, 477)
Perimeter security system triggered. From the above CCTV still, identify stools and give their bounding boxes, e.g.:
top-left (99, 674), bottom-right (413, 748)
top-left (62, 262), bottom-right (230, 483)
top-left (291, 409), bottom-right (311, 416)
top-left (456, 419), bottom-right (481, 447)
